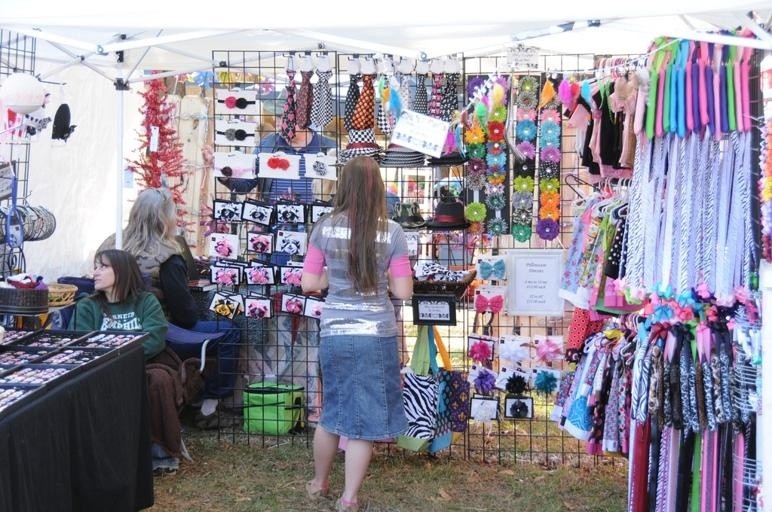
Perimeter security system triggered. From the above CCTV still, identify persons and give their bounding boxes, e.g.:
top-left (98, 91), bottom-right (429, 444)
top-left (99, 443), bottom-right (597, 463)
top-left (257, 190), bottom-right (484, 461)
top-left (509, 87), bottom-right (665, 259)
top-left (301, 155), bottom-right (414, 512)
top-left (217, 95), bottom-right (338, 272)
top-left (95, 187), bottom-right (242, 429)
top-left (67, 248), bottom-right (185, 478)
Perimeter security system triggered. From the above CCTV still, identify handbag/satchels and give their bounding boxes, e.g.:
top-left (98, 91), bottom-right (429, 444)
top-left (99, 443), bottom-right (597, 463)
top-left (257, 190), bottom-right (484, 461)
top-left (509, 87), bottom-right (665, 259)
top-left (397, 369), bottom-right (469, 454)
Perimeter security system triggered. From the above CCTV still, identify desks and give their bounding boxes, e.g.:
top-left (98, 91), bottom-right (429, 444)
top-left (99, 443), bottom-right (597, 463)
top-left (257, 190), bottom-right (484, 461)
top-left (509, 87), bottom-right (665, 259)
top-left (0, 342), bottom-right (144, 512)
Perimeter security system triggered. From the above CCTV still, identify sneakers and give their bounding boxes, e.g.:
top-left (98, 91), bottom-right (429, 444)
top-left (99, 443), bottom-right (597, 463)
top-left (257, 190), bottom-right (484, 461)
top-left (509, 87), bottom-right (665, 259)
top-left (306, 478), bottom-right (328, 499)
top-left (153, 466), bottom-right (176, 477)
top-left (336, 497), bottom-right (360, 512)
top-left (196, 409), bottom-right (238, 431)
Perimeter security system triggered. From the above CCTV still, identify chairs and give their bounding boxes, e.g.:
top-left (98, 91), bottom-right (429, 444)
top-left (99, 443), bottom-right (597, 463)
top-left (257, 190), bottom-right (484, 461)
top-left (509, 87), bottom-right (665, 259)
top-left (145, 321), bottom-right (224, 463)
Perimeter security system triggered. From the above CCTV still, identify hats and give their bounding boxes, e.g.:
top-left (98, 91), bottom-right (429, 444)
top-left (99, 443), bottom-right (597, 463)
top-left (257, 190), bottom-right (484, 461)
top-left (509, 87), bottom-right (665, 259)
top-left (391, 202), bottom-right (427, 230)
top-left (428, 203), bottom-right (473, 230)
top-left (427, 152), bottom-right (468, 165)
top-left (339, 128), bottom-right (426, 168)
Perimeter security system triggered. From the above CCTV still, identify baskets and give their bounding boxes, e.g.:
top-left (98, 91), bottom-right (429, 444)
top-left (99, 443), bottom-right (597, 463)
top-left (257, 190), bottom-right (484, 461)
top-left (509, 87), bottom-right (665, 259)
top-left (45, 284), bottom-right (78, 306)
